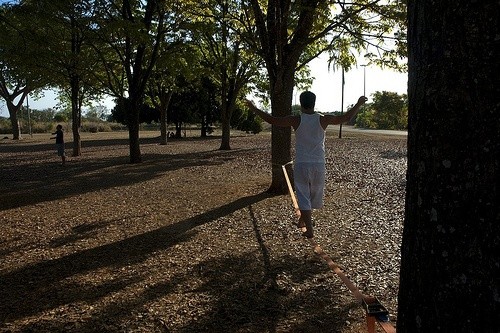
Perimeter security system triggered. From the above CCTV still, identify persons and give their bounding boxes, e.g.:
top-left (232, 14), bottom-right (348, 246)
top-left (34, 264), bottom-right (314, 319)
top-left (50, 123), bottom-right (66, 166)
top-left (167, 130), bottom-right (175, 137)
top-left (243, 90), bottom-right (368, 237)
